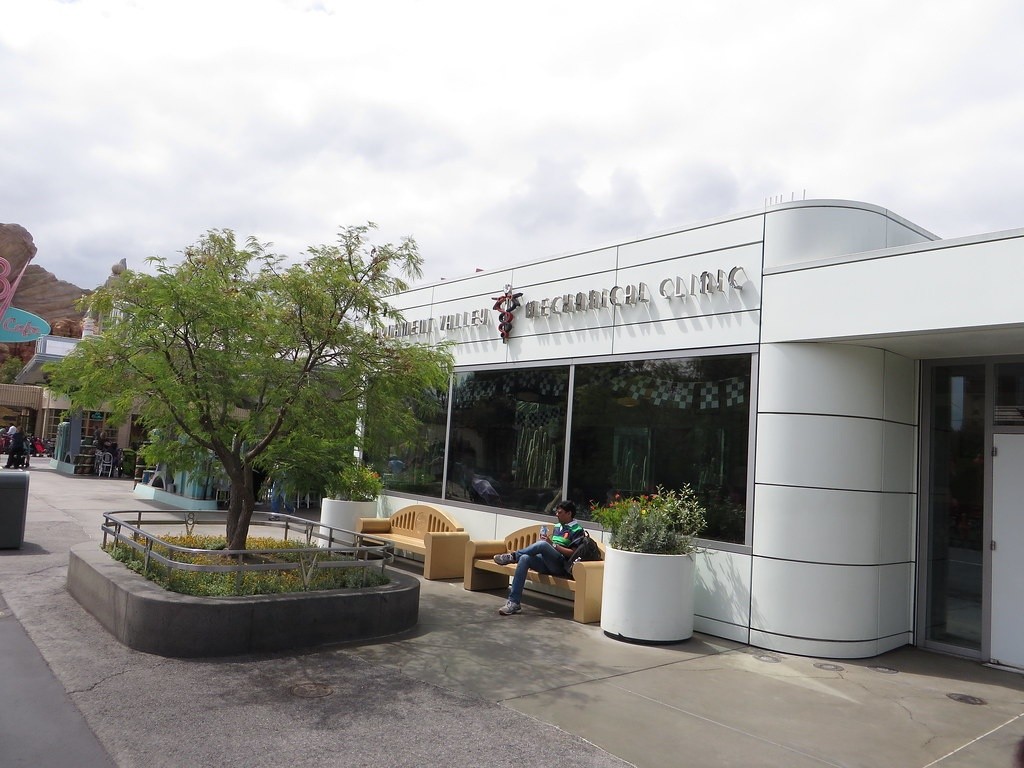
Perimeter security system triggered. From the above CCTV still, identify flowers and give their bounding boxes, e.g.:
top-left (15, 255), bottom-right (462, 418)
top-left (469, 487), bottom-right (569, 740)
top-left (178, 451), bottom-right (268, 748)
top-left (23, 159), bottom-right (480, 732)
top-left (589, 483), bottom-right (718, 556)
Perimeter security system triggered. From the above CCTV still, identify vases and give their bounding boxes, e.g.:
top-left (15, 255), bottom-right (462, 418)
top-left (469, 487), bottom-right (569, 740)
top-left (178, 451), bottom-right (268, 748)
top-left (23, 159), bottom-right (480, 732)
top-left (599, 546), bottom-right (697, 642)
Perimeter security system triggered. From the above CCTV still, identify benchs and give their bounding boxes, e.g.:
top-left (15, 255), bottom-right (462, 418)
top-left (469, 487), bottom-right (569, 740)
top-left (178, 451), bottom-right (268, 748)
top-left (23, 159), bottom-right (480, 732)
top-left (464, 525), bottom-right (604, 624)
top-left (355, 504), bottom-right (470, 580)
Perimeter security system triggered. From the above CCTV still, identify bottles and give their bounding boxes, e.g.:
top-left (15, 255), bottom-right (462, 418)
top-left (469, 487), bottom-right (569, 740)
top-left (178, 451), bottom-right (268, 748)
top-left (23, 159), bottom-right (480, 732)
top-left (540, 526), bottom-right (546, 535)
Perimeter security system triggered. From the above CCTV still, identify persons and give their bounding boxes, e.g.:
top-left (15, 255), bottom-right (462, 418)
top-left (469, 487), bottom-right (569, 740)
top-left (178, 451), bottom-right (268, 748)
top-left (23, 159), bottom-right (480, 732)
top-left (94, 433), bottom-right (145, 476)
top-left (269, 468), bottom-right (296, 521)
top-left (493, 501), bottom-right (584, 615)
top-left (93, 425), bottom-right (101, 436)
top-left (0, 422), bottom-right (52, 469)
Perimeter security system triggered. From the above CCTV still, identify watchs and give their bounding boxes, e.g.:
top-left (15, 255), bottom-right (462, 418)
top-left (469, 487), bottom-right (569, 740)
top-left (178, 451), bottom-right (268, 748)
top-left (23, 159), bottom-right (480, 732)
top-left (552, 544), bottom-right (557, 548)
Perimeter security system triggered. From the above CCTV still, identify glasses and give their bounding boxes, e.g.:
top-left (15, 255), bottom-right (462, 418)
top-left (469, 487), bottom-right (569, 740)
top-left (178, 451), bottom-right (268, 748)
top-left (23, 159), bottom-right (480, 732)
top-left (555, 510), bottom-right (566, 514)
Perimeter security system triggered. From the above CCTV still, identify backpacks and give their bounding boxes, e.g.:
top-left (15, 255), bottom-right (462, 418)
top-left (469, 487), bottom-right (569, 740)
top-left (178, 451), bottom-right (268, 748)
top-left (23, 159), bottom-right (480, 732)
top-left (567, 530), bottom-right (601, 575)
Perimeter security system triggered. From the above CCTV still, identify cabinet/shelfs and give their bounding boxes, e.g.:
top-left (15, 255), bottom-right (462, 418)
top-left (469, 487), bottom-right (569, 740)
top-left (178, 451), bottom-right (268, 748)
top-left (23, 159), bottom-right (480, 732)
top-left (47, 409), bottom-right (119, 475)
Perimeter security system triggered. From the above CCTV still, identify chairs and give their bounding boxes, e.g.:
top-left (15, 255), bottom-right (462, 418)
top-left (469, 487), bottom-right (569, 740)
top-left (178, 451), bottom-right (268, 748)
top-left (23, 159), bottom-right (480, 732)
top-left (94, 448), bottom-right (114, 477)
top-left (216, 475), bottom-right (323, 508)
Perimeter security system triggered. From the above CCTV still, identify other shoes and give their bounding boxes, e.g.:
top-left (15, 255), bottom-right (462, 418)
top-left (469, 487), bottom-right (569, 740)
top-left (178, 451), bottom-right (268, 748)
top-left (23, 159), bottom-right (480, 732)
top-left (288, 507), bottom-right (296, 521)
top-left (269, 516), bottom-right (280, 521)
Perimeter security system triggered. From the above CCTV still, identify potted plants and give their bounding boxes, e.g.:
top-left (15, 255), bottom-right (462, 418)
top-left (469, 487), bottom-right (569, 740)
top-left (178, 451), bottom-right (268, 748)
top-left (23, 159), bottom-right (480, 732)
top-left (318, 458), bottom-right (385, 553)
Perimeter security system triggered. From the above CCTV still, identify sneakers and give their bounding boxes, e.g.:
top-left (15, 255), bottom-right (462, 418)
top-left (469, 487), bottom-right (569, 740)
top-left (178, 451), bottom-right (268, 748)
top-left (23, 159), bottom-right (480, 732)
top-left (498, 601), bottom-right (522, 616)
top-left (493, 553), bottom-right (519, 566)
